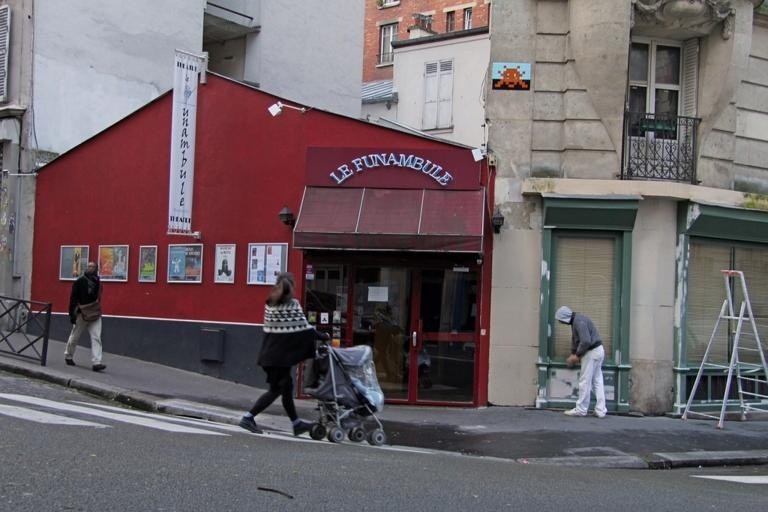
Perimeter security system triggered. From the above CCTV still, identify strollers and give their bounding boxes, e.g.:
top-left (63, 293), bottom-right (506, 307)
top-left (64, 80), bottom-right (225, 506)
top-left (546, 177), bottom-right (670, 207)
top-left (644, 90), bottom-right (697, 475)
top-left (295, 328), bottom-right (388, 447)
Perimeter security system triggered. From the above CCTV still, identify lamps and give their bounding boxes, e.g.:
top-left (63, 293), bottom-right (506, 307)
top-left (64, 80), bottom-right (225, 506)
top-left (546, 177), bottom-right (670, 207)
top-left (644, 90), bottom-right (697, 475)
top-left (470, 147), bottom-right (488, 163)
top-left (266, 98), bottom-right (307, 120)
top-left (488, 208), bottom-right (504, 235)
top-left (275, 202), bottom-right (298, 230)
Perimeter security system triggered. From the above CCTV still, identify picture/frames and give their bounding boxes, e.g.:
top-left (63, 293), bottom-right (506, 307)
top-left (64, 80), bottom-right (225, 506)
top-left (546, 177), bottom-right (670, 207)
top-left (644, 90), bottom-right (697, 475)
top-left (57, 240), bottom-right (289, 287)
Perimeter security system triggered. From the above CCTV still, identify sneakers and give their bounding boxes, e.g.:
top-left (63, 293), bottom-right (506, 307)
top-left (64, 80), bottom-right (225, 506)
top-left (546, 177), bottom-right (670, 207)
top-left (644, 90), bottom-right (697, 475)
top-left (592, 409), bottom-right (606, 419)
top-left (92, 363), bottom-right (107, 372)
top-left (292, 420), bottom-right (317, 437)
top-left (238, 415), bottom-right (264, 434)
top-left (562, 407), bottom-right (588, 418)
top-left (64, 358), bottom-right (76, 367)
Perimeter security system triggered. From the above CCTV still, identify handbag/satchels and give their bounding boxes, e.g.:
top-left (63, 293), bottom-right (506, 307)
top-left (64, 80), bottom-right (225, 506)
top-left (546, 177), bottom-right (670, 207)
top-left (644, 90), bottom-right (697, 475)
top-left (78, 299), bottom-right (103, 323)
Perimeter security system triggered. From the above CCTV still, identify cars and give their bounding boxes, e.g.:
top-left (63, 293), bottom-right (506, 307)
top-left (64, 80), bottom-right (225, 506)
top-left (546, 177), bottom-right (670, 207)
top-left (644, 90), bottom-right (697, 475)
top-left (305, 286), bottom-right (474, 390)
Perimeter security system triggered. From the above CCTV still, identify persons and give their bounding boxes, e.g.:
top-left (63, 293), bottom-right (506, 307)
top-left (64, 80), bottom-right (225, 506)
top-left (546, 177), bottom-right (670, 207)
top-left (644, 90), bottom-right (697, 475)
top-left (65, 262), bottom-right (108, 370)
top-left (75, 249), bottom-right (81, 270)
top-left (241, 274), bottom-right (328, 437)
top-left (555, 305), bottom-right (609, 418)
top-left (112, 249), bottom-right (125, 273)
top-left (369, 303), bottom-right (400, 381)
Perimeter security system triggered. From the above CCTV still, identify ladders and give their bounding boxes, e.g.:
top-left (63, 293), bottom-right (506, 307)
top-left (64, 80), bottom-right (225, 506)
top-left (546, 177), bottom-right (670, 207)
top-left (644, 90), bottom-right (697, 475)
top-left (681, 270), bottom-right (768, 429)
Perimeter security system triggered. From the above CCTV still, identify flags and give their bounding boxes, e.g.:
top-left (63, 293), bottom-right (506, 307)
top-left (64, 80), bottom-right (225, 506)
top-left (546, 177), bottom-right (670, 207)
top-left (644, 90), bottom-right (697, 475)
top-left (165, 46), bottom-right (205, 237)
